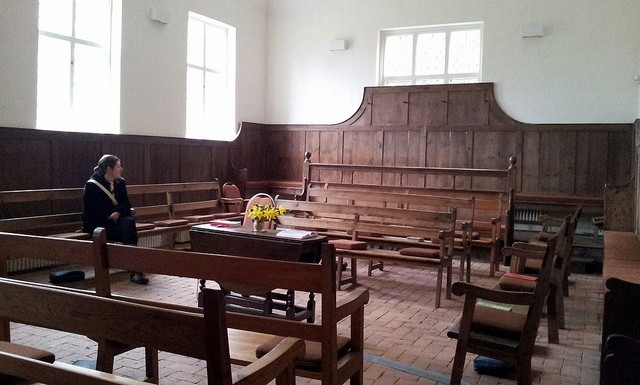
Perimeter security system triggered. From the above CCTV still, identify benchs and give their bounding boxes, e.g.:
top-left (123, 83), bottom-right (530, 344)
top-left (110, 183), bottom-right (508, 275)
top-left (446, 216), bottom-right (573, 385)
top-left (1, 232), bottom-right (369, 385)
top-left (245, 181), bottom-right (604, 266)
top-left (270, 195), bottom-right (457, 308)
top-left (512, 205), bottom-right (583, 296)
top-left (280, 177), bottom-right (516, 278)
top-left (0, 177), bottom-right (243, 278)
top-left (307, 188), bottom-right (475, 282)
top-left (0, 277), bottom-right (305, 384)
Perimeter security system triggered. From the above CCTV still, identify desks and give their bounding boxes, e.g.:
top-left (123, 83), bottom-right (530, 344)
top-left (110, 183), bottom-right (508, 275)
top-left (189, 220), bottom-right (328, 263)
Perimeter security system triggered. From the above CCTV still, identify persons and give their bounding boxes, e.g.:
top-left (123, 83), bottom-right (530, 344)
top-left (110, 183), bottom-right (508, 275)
top-left (81, 154), bottom-right (150, 284)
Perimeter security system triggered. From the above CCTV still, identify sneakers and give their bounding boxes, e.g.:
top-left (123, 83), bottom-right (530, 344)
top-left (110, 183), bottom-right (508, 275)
top-left (130, 273), bottom-right (149, 284)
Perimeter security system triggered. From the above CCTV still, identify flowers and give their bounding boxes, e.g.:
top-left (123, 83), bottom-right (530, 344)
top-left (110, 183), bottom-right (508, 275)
top-left (246, 203), bottom-right (286, 233)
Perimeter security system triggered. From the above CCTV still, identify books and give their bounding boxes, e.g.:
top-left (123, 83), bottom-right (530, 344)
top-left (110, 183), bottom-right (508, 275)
top-left (276, 227), bottom-right (319, 239)
top-left (207, 218), bottom-right (242, 227)
